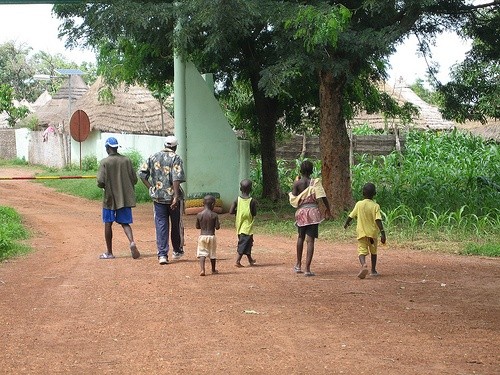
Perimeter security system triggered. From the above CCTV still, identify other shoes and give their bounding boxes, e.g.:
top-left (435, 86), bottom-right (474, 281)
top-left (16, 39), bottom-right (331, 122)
top-left (293, 264), bottom-right (316, 277)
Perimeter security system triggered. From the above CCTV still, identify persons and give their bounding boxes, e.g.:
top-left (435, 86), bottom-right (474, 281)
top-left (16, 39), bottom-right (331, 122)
top-left (344, 183), bottom-right (386, 278)
top-left (139, 136), bottom-right (188, 265)
top-left (289, 160), bottom-right (333, 275)
top-left (230, 178), bottom-right (257, 268)
top-left (96, 137), bottom-right (140, 260)
top-left (194, 195), bottom-right (221, 276)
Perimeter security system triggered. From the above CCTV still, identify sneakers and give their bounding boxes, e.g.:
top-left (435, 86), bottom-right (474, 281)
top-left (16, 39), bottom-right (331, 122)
top-left (158, 251), bottom-right (184, 264)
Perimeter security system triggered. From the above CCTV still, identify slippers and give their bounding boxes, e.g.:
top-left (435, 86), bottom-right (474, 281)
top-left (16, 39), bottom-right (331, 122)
top-left (129, 245), bottom-right (140, 259)
top-left (358, 264), bottom-right (381, 279)
top-left (98, 252), bottom-right (116, 259)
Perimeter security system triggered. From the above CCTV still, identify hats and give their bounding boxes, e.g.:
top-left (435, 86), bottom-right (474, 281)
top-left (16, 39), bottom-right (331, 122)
top-left (105, 137), bottom-right (122, 148)
top-left (164, 135), bottom-right (179, 147)
